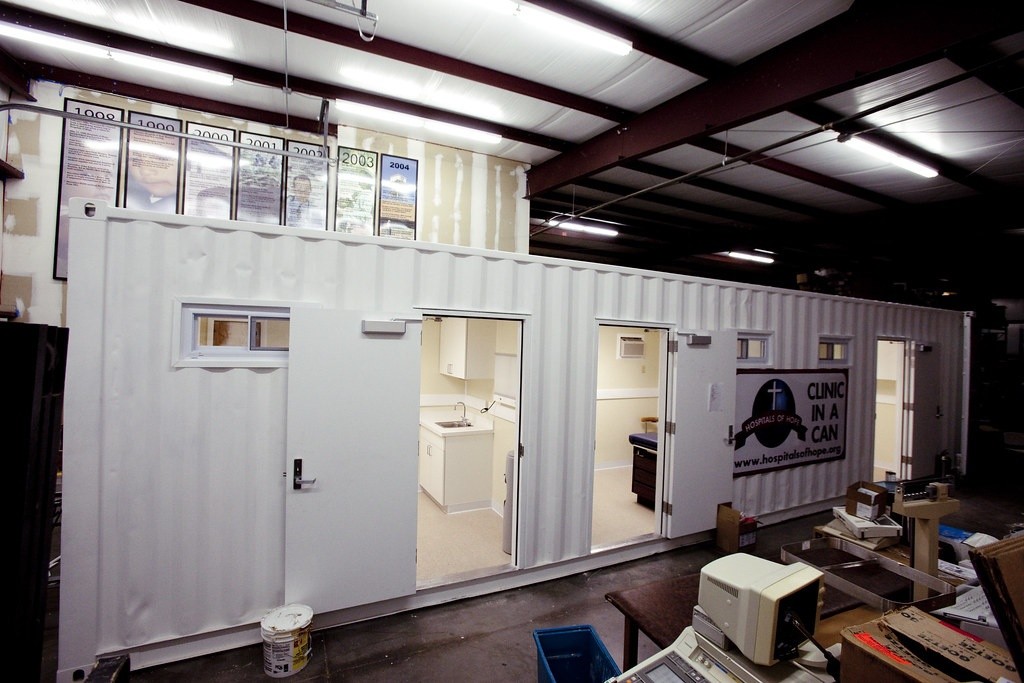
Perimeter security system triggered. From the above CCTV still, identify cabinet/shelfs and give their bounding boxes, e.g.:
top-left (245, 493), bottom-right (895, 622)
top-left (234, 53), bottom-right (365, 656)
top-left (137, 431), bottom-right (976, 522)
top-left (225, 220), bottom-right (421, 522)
top-left (629, 431), bottom-right (657, 513)
top-left (418, 425), bottom-right (494, 515)
top-left (438, 317), bottom-right (496, 380)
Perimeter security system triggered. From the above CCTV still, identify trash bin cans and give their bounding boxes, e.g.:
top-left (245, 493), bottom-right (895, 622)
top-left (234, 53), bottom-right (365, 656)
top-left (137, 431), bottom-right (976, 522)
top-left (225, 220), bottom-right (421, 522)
top-left (501, 449), bottom-right (514, 555)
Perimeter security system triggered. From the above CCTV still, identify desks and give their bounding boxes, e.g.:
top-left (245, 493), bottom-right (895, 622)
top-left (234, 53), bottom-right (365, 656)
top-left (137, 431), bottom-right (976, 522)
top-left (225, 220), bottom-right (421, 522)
top-left (602, 553), bottom-right (912, 675)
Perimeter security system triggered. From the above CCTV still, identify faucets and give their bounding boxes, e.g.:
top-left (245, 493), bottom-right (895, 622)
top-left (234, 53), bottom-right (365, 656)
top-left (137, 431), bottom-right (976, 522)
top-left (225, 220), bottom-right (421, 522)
top-left (454, 402), bottom-right (469, 422)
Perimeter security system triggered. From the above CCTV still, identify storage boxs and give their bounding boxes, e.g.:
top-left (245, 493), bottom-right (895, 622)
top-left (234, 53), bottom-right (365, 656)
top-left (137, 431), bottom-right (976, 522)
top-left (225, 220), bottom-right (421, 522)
top-left (532, 627), bottom-right (622, 683)
top-left (845, 479), bottom-right (889, 522)
top-left (837, 605), bottom-right (1024, 683)
top-left (716, 501), bottom-right (759, 556)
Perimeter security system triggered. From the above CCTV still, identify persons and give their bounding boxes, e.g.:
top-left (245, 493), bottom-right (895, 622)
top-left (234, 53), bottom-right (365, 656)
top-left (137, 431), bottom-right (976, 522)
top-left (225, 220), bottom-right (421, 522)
top-left (387, 220), bottom-right (392, 235)
top-left (287, 175), bottom-right (325, 231)
top-left (253, 154), bottom-right (278, 169)
top-left (126, 129), bottom-right (179, 214)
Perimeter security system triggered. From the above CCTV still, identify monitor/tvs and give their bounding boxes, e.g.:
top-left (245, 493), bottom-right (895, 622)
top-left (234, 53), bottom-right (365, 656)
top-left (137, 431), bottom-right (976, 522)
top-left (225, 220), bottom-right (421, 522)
top-left (698, 552), bottom-right (824, 666)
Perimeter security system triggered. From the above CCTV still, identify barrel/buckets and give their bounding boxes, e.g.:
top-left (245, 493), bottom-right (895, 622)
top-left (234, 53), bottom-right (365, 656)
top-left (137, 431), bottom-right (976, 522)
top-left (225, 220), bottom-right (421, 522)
top-left (261, 604), bottom-right (314, 678)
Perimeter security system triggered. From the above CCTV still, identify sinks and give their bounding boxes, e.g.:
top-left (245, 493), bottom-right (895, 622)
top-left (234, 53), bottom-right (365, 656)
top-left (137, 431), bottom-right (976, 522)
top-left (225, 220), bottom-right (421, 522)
top-left (435, 421), bottom-right (473, 428)
